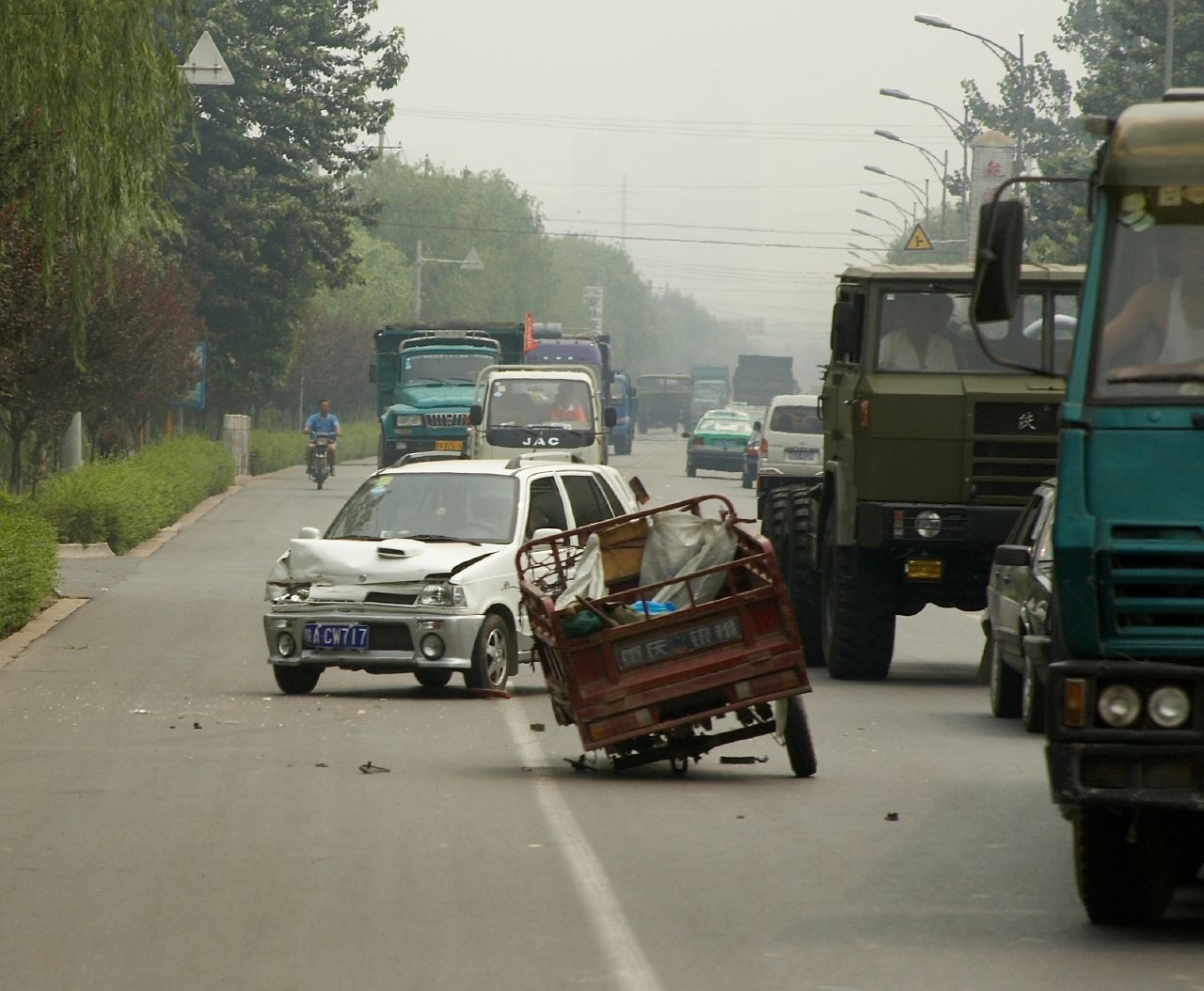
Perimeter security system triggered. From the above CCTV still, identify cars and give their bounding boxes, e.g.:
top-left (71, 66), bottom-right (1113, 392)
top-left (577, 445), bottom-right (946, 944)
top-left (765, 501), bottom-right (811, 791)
top-left (682, 407), bottom-right (753, 479)
top-left (982, 473), bottom-right (1061, 733)
top-left (738, 420), bottom-right (768, 490)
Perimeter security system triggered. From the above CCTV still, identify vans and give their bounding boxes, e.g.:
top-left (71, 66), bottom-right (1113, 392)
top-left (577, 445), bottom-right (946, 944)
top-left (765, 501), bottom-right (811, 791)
top-left (750, 393), bottom-right (826, 490)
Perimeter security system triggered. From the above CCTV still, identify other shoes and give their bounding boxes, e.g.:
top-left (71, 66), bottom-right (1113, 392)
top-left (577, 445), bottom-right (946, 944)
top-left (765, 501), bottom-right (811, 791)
top-left (306, 466), bottom-right (312, 476)
top-left (330, 466), bottom-right (335, 477)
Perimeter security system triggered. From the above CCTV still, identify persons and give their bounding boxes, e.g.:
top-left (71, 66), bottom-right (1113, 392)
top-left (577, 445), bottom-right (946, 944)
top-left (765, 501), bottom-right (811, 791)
top-left (1096, 227), bottom-right (1203, 371)
top-left (302, 398), bottom-right (340, 476)
top-left (879, 294), bottom-right (956, 373)
top-left (547, 383), bottom-right (585, 422)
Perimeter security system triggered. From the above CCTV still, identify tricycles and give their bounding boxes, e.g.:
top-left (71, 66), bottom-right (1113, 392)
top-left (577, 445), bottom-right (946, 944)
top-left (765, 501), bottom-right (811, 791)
top-left (513, 490), bottom-right (826, 784)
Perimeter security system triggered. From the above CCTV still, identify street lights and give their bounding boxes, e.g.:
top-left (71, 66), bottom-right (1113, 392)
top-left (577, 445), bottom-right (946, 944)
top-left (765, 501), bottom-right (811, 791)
top-left (912, 11), bottom-right (1030, 199)
top-left (863, 164), bottom-right (931, 249)
top-left (875, 128), bottom-right (951, 262)
top-left (863, 189), bottom-right (920, 230)
top-left (880, 82), bottom-right (970, 252)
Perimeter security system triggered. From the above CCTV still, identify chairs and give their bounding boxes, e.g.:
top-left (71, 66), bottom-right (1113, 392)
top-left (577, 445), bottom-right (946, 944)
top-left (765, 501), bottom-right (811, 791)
top-left (492, 392), bottom-right (539, 426)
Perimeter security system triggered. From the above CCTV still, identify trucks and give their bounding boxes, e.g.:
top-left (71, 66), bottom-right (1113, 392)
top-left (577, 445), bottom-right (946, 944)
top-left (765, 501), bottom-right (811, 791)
top-left (750, 260), bottom-right (1090, 679)
top-left (365, 320), bottom-right (803, 470)
top-left (969, 86), bottom-right (1204, 931)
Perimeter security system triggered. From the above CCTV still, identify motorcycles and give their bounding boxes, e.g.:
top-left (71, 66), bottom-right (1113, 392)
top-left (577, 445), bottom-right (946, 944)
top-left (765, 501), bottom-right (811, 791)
top-left (306, 427), bottom-right (339, 490)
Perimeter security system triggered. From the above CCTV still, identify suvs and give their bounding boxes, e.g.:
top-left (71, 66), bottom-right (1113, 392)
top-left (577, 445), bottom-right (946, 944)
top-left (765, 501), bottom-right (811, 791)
top-left (262, 449), bottom-right (642, 699)
